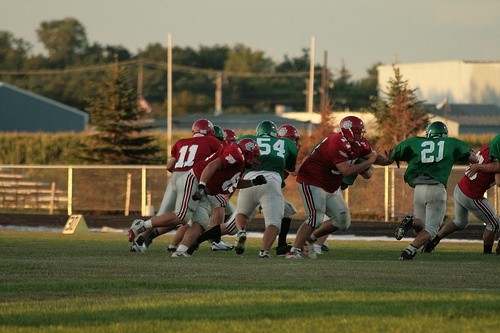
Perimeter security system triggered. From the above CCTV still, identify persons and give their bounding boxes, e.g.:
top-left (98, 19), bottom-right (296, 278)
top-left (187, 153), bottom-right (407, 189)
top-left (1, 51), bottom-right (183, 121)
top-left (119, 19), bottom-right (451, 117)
top-left (127, 119), bottom-right (303, 258)
top-left (287, 116), bottom-right (395, 258)
top-left (392, 121), bottom-right (479, 260)
top-left (425, 133), bottom-right (500, 255)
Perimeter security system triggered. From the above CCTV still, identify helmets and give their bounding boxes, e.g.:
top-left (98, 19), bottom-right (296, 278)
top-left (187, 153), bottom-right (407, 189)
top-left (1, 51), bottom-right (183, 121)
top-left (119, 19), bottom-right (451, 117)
top-left (213, 125), bottom-right (226, 141)
top-left (192, 119), bottom-right (215, 137)
top-left (277, 124), bottom-right (300, 140)
top-left (339, 116), bottom-right (366, 146)
top-left (256, 120), bottom-right (278, 138)
top-left (426, 121), bottom-right (449, 138)
top-left (222, 128), bottom-right (237, 144)
top-left (236, 137), bottom-right (260, 170)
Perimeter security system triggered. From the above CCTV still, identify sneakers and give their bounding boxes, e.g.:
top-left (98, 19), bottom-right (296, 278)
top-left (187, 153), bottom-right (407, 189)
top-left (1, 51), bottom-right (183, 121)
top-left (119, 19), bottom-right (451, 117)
top-left (421, 240), bottom-right (435, 252)
top-left (400, 249), bottom-right (417, 261)
top-left (128, 219), bottom-right (330, 259)
top-left (394, 215), bottom-right (414, 240)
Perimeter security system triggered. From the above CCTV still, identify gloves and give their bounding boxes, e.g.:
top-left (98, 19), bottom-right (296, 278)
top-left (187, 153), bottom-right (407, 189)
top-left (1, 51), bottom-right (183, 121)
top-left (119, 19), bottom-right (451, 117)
top-left (192, 184), bottom-right (206, 201)
top-left (253, 174), bottom-right (266, 186)
